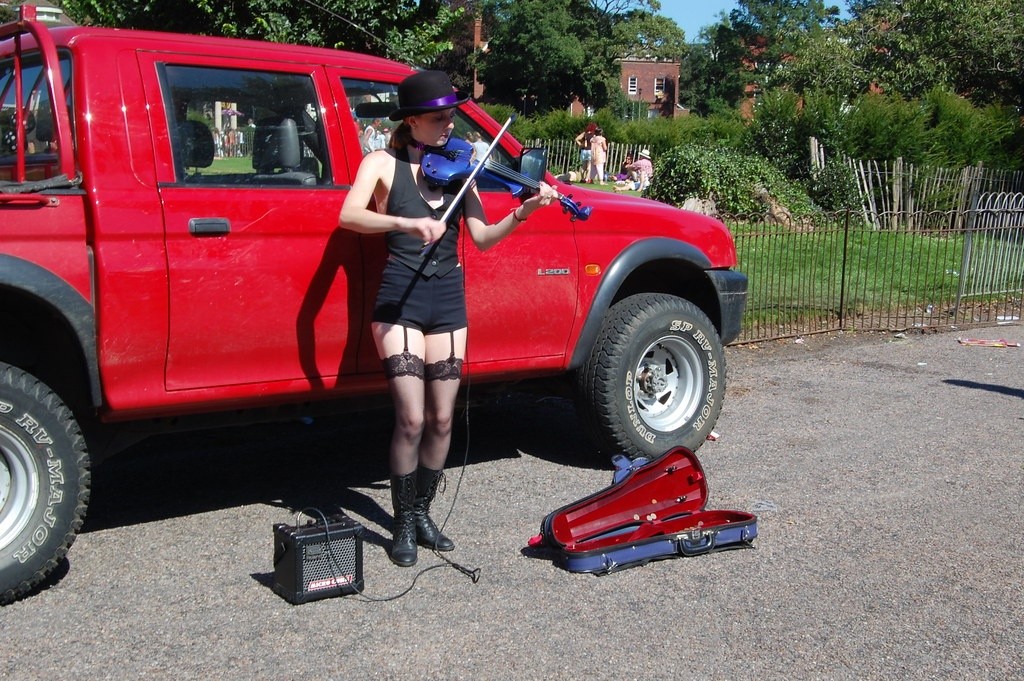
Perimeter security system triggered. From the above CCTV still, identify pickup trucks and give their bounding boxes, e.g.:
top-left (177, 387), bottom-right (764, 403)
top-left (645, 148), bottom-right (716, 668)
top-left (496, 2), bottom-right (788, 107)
top-left (0, 0), bottom-right (749, 599)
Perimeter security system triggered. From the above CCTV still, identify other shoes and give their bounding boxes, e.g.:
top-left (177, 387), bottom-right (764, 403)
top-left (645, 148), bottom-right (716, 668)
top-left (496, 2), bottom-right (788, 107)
top-left (600, 182), bottom-right (608, 185)
top-left (580, 179), bottom-right (593, 184)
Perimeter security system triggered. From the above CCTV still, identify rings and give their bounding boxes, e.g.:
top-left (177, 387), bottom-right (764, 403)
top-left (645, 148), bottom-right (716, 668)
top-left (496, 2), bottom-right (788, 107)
top-left (547, 195), bottom-right (552, 199)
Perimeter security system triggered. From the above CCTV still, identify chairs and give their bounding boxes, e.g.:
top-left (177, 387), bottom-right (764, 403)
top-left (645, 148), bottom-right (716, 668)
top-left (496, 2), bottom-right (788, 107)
top-left (251, 117), bottom-right (316, 186)
top-left (178, 120), bottom-right (215, 183)
top-left (36, 100), bottom-right (55, 151)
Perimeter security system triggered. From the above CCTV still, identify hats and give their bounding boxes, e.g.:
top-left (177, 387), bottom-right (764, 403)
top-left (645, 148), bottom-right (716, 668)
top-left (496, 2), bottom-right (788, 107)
top-left (638, 149), bottom-right (651, 159)
top-left (390, 69), bottom-right (470, 121)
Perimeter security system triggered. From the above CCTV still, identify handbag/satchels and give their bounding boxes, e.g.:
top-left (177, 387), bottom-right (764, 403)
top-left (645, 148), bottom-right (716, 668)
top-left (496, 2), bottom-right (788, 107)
top-left (580, 140), bottom-right (587, 147)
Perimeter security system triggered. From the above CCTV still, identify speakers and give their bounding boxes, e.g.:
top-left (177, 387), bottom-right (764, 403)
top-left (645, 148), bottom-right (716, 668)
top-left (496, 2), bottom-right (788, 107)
top-left (273, 513), bottom-right (364, 605)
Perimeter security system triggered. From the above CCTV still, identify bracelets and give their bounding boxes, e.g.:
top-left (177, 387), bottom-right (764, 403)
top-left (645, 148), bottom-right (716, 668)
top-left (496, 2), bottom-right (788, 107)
top-left (510, 209), bottom-right (527, 222)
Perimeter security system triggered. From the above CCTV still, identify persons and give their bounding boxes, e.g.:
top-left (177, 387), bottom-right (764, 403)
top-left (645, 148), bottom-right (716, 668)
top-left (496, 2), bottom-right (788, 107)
top-left (212, 127), bottom-right (244, 158)
top-left (608, 149), bottom-right (653, 194)
top-left (589, 127), bottom-right (609, 185)
top-left (362, 118), bottom-right (382, 156)
top-left (464, 131), bottom-right (492, 165)
top-left (353, 117), bottom-right (360, 133)
top-left (338, 69), bottom-right (558, 567)
top-left (575, 122), bottom-right (598, 184)
top-left (356, 126), bottom-right (391, 153)
top-left (247, 119), bottom-right (256, 127)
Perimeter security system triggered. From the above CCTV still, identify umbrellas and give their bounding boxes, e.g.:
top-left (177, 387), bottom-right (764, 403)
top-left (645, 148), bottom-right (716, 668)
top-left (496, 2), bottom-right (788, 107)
top-left (221, 109), bottom-right (245, 127)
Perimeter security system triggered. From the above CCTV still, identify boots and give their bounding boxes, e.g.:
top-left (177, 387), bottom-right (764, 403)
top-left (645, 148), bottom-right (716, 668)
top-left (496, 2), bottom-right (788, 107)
top-left (389, 465), bottom-right (455, 566)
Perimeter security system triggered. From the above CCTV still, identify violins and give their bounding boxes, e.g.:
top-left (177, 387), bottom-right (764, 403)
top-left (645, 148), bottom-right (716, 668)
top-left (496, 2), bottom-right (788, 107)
top-left (419, 135), bottom-right (595, 226)
